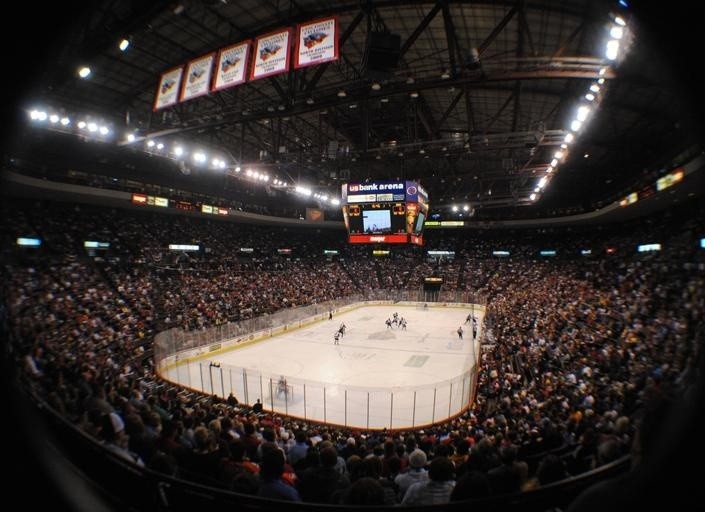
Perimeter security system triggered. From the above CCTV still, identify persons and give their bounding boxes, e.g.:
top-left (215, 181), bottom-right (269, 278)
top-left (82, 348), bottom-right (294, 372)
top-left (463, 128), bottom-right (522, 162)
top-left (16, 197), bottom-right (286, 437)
top-left (0, 172), bottom-right (704, 512)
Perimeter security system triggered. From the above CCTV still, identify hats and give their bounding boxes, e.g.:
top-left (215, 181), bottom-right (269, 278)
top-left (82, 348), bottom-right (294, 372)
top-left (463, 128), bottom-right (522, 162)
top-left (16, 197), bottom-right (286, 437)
top-left (347, 437), bottom-right (355, 445)
top-left (409, 449), bottom-right (426, 467)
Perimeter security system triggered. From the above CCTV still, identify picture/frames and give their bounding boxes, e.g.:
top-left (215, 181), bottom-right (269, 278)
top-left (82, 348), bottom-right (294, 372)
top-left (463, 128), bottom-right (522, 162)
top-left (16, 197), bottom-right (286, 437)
top-left (152, 15), bottom-right (340, 113)
